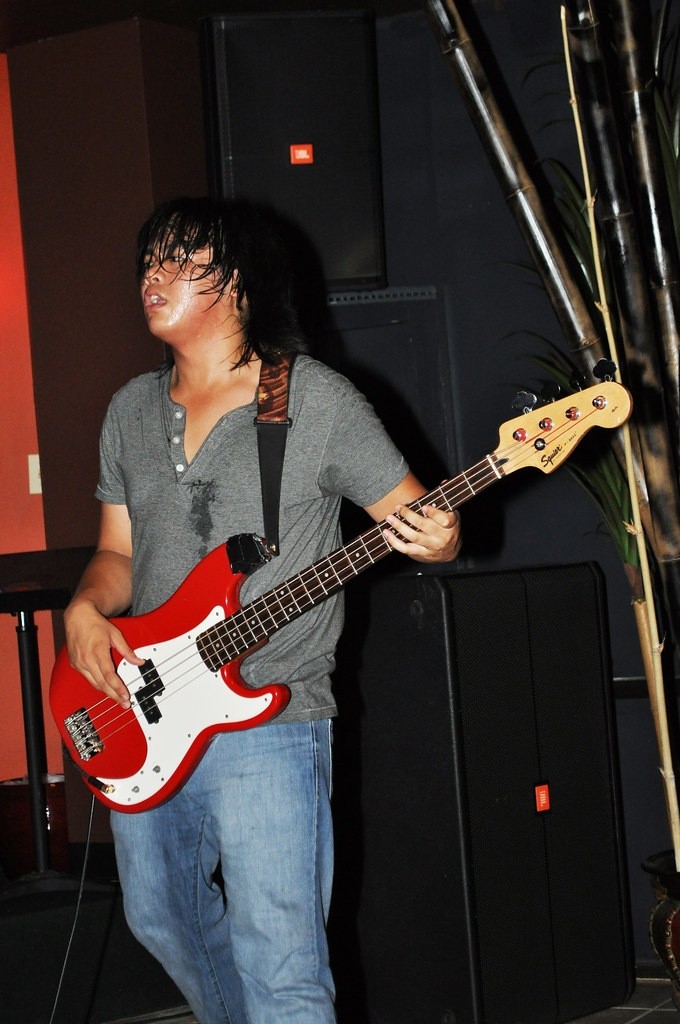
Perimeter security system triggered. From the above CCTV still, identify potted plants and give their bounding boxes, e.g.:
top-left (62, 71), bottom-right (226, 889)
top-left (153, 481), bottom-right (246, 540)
top-left (521, 104), bottom-right (680, 1011)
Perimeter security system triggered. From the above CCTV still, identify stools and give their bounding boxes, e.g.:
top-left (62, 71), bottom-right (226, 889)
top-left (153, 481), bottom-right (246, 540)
top-left (0, 546), bottom-right (119, 905)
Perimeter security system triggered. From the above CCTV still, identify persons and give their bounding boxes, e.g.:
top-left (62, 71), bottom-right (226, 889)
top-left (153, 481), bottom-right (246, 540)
top-left (63, 197), bottom-right (461, 1024)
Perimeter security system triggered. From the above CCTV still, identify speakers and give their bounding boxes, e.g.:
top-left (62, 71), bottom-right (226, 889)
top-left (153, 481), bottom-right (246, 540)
top-left (325, 283), bottom-right (503, 574)
top-left (329, 560), bottom-right (636, 1024)
top-left (212, 9), bottom-right (387, 291)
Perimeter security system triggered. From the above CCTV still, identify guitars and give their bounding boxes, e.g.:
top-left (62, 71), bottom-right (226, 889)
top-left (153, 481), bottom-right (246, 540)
top-left (49, 356), bottom-right (632, 818)
